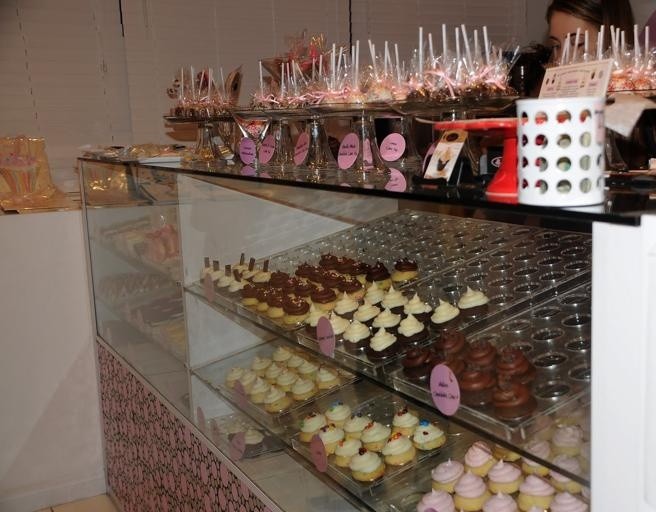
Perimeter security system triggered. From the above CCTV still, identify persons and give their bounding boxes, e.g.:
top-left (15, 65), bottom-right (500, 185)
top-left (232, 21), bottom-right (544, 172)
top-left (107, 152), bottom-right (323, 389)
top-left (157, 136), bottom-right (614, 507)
top-left (546, 0), bottom-right (635, 66)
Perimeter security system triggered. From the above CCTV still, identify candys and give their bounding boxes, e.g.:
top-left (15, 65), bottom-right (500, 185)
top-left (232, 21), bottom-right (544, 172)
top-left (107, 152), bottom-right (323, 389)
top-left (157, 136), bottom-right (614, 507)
top-left (249, 23), bottom-right (520, 113)
top-left (175, 65), bottom-right (230, 120)
top-left (542, 24), bottom-right (656, 98)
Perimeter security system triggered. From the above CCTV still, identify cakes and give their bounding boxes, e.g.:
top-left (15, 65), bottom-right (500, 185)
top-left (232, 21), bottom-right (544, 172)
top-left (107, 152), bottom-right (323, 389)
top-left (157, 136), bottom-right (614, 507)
top-left (298, 407), bottom-right (328, 444)
top-left (551, 422), bottom-right (583, 459)
top-left (402, 347), bottom-right (441, 383)
top-left (239, 368), bottom-right (257, 395)
top-left (210, 259), bottom-right (222, 287)
top-left (340, 275), bottom-right (365, 301)
top-left (458, 285), bottom-right (491, 317)
top-left (295, 277), bottom-right (318, 298)
top-left (217, 264), bottom-right (233, 294)
top-left (430, 457), bottom-right (465, 494)
top-left (199, 255), bottom-right (213, 283)
top-left (232, 252), bottom-right (248, 274)
top-left (360, 421), bottom-right (391, 452)
top-left (255, 286), bottom-right (269, 312)
top-left (249, 375), bottom-right (270, 403)
top-left (276, 368), bottom-right (300, 392)
top-left (335, 438), bottom-right (363, 467)
top-left (283, 274), bottom-right (303, 294)
top-left (296, 358), bottom-right (320, 380)
top-left (381, 283), bottom-right (406, 313)
top-left (241, 283), bottom-right (259, 306)
top-left (392, 257), bottom-right (419, 281)
top-left (318, 254), bottom-right (338, 266)
top-left (398, 312), bottom-right (429, 342)
top-left (335, 290), bottom-right (359, 319)
top-left (316, 364), bottom-right (341, 390)
top-left (413, 420), bottom-right (446, 450)
top-left (367, 326), bottom-right (400, 360)
top-left (295, 260), bottom-right (312, 278)
top-left (265, 362), bottom-right (284, 384)
top-left (349, 262), bottom-right (372, 284)
top-left (306, 302), bottom-right (328, 337)
top-left (439, 354), bottom-right (464, 376)
top-left (287, 350), bottom-right (303, 371)
top-left (364, 278), bottom-right (384, 308)
top-left (342, 319), bottom-right (372, 350)
top-left (366, 260), bottom-right (392, 290)
top-left (517, 473), bottom-right (556, 511)
top-left (343, 409), bottom-right (372, 439)
top-left (434, 328), bottom-right (468, 355)
top-left (481, 491), bottom-right (519, 512)
top-left (455, 369), bottom-right (496, 410)
top-left (264, 384), bottom-right (291, 411)
top-left (460, 337), bottom-right (499, 375)
top-left (240, 429), bottom-right (262, 457)
top-left (309, 264), bottom-right (324, 282)
top-left (392, 407), bottom-right (419, 437)
top-left (265, 286), bottom-right (286, 317)
top-left (352, 297), bottom-right (381, 326)
top-left (229, 268), bottom-right (250, 301)
top-left (371, 305), bottom-right (402, 336)
top-left (262, 430), bottom-right (276, 449)
top-left (403, 292), bottom-right (433, 321)
top-left (282, 297), bottom-right (309, 323)
top-left (292, 376), bottom-right (317, 400)
top-left (226, 365), bottom-right (243, 387)
top-left (243, 257), bottom-right (257, 281)
top-left (251, 350), bottom-right (271, 373)
top-left (416, 488), bottom-right (456, 512)
top-left (491, 374), bottom-right (537, 424)
top-left (521, 439), bottom-right (551, 476)
top-left (325, 398), bottom-right (352, 427)
top-left (310, 286), bottom-right (338, 311)
top-left (318, 422), bottom-right (344, 455)
top-left (463, 440), bottom-right (496, 477)
top-left (327, 310), bottom-right (350, 341)
top-left (549, 491), bottom-right (589, 512)
top-left (495, 344), bottom-right (537, 387)
top-left (272, 342), bottom-right (294, 364)
top-left (269, 270), bottom-right (288, 287)
top-left (430, 298), bottom-right (461, 332)
top-left (454, 470), bottom-right (490, 511)
top-left (492, 441), bottom-right (520, 461)
top-left (252, 258), bottom-right (274, 288)
top-left (320, 268), bottom-right (339, 287)
top-left (547, 455), bottom-right (584, 493)
top-left (334, 256), bottom-right (358, 278)
top-left (381, 431), bottom-right (417, 466)
top-left (487, 459), bottom-right (523, 494)
top-left (348, 447), bottom-right (385, 482)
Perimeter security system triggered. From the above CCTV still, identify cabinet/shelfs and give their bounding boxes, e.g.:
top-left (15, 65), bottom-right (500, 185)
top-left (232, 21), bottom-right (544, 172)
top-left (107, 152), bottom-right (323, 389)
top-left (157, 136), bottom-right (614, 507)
top-left (78, 157), bottom-right (655, 511)
